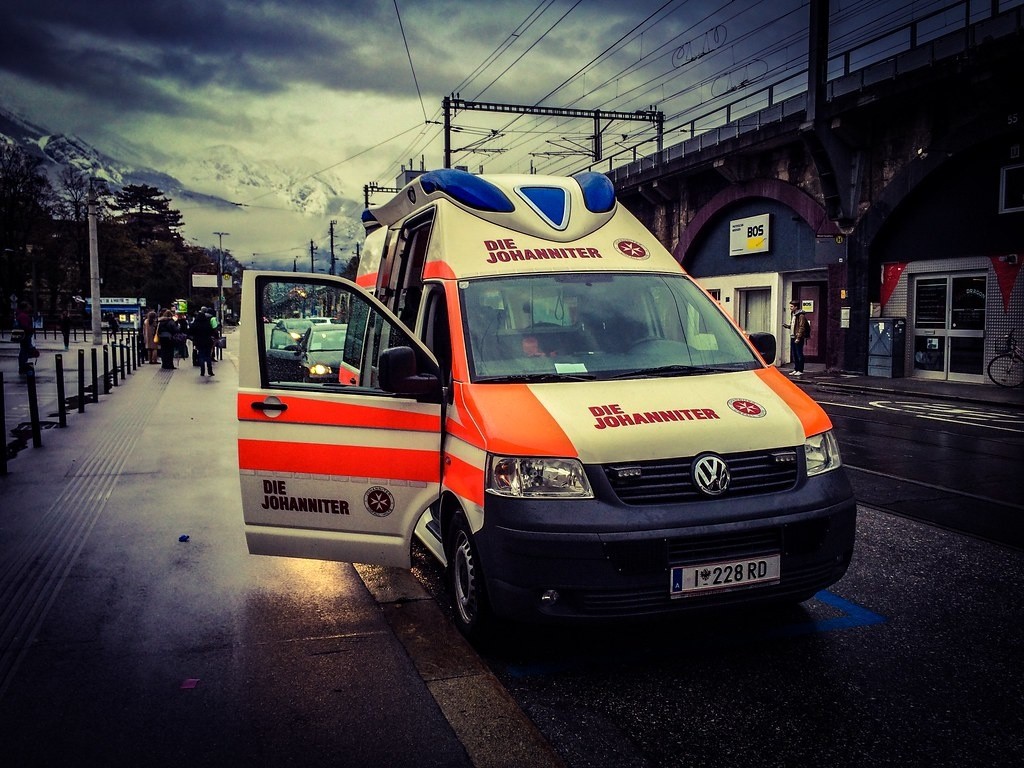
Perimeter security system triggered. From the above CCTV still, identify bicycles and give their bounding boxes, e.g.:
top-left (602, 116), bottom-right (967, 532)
top-left (987, 329), bottom-right (1024, 389)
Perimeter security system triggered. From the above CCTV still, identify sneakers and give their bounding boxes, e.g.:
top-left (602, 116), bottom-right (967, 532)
top-left (794, 371), bottom-right (803, 375)
top-left (788, 371), bottom-right (796, 375)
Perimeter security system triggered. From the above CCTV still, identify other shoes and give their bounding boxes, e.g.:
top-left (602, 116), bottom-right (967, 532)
top-left (149, 362), bottom-right (161, 364)
top-left (212, 358), bottom-right (217, 362)
top-left (200, 372), bottom-right (215, 376)
top-left (162, 366), bottom-right (177, 369)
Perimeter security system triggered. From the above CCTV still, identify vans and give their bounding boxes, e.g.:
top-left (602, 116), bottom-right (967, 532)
top-left (239, 171), bottom-right (857, 640)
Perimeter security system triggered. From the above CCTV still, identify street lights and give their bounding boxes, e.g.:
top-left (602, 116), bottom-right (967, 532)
top-left (212, 232), bottom-right (231, 329)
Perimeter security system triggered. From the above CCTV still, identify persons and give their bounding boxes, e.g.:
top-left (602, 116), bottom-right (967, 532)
top-left (156, 310), bottom-right (177, 370)
top-left (142, 304), bottom-right (219, 364)
top-left (782, 299), bottom-right (810, 376)
top-left (108, 313), bottom-right (119, 339)
top-left (14, 302), bottom-right (37, 375)
top-left (59, 310), bottom-right (73, 350)
top-left (291, 311), bottom-right (301, 320)
top-left (188, 311), bottom-right (221, 376)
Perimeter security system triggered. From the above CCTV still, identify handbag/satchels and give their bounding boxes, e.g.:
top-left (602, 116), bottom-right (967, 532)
top-left (215, 337), bottom-right (226, 348)
top-left (154, 333), bottom-right (159, 343)
top-left (10, 330), bottom-right (25, 341)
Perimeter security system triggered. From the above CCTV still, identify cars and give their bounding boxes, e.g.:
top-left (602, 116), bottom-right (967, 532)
top-left (267, 324), bottom-right (349, 384)
top-left (272, 318), bottom-right (316, 341)
top-left (307, 316), bottom-right (339, 325)
